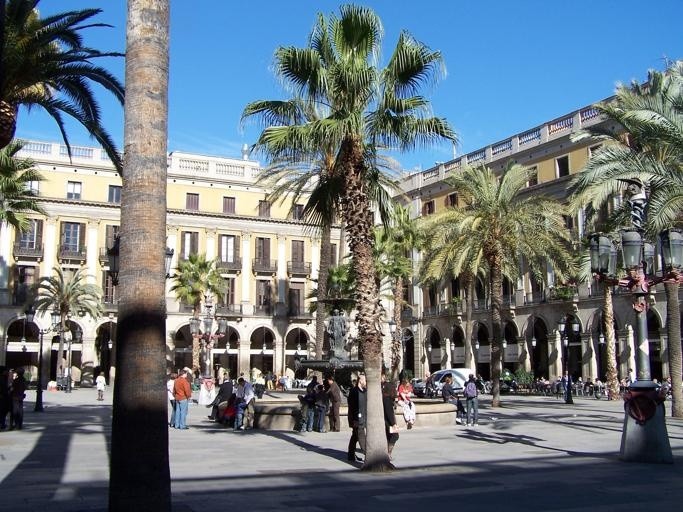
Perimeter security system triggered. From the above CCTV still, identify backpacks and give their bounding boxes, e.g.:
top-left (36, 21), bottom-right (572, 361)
top-left (464, 381), bottom-right (477, 398)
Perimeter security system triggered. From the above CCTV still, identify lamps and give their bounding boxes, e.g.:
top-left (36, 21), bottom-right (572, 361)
top-left (17, 325), bottom-right (304, 354)
top-left (424, 325), bottom-right (605, 354)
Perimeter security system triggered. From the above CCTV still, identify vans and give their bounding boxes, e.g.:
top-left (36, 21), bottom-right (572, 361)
top-left (412, 367), bottom-right (477, 400)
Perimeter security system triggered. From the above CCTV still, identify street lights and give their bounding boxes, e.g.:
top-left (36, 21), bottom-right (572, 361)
top-left (191, 308), bottom-right (227, 410)
top-left (555, 311), bottom-right (582, 404)
top-left (21, 300), bottom-right (63, 413)
top-left (588, 220), bottom-right (682, 468)
top-left (104, 223), bottom-right (175, 288)
top-left (389, 315), bottom-right (419, 387)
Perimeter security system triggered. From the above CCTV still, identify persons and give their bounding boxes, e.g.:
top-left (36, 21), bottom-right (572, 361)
top-left (262, 371), bottom-right (289, 392)
top-left (511, 379), bottom-right (517, 394)
top-left (298, 376), bottom-right (341, 432)
top-left (96, 372), bottom-right (107, 401)
top-left (206, 372), bottom-right (255, 431)
top-left (536, 368), bottom-right (672, 402)
top-left (442, 374), bottom-right (479, 427)
top-left (1, 366), bottom-right (26, 431)
top-left (382, 376), bottom-right (416, 461)
top-left (167, 368), bottom-right (191, 430)
top-left (425, 376), bottom-right (437, 398)
top-left (348, 375), bottom-right (367, 463)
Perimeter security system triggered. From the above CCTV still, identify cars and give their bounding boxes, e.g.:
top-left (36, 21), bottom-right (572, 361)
top-left (410, 378), bottom-right (423, 388)
